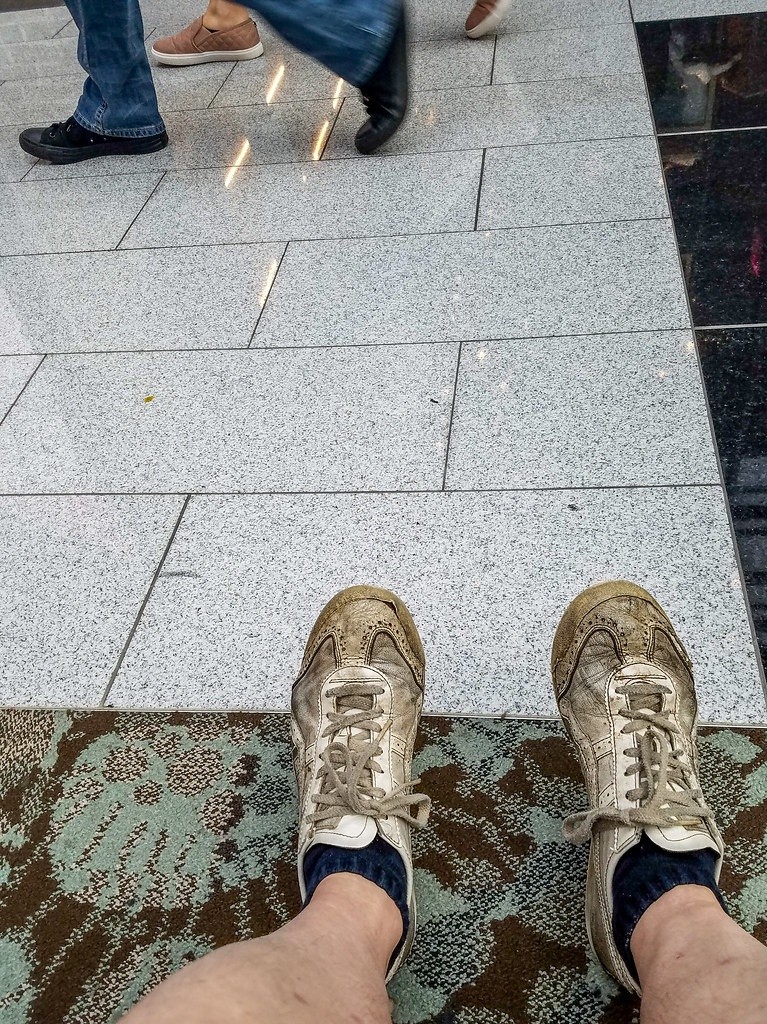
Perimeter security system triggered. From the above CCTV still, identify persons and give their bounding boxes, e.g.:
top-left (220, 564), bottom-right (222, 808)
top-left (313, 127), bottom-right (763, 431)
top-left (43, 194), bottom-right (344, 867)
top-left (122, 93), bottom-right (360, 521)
top-left (19, 0), bottom-right (410, 164)
top-left (151, 0), bottom-right (510, 66)
top-left (118, 586), bottom-right (767, 1024)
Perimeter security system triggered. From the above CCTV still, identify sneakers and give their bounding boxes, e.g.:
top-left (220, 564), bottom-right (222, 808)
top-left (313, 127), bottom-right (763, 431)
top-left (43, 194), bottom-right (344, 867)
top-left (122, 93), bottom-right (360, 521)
top-left (19, 115), bottom-right (169, 163)
top-left (151, 16), bottom-right (264, 66)
top-left (464, 0), bottom-right (510, 38)
top-left (354, 3), bottom-right (409, 154)
top-left (551, 580), bottom-right (725, 999)
top-left (291, 586), bottom-right (431, 986)
